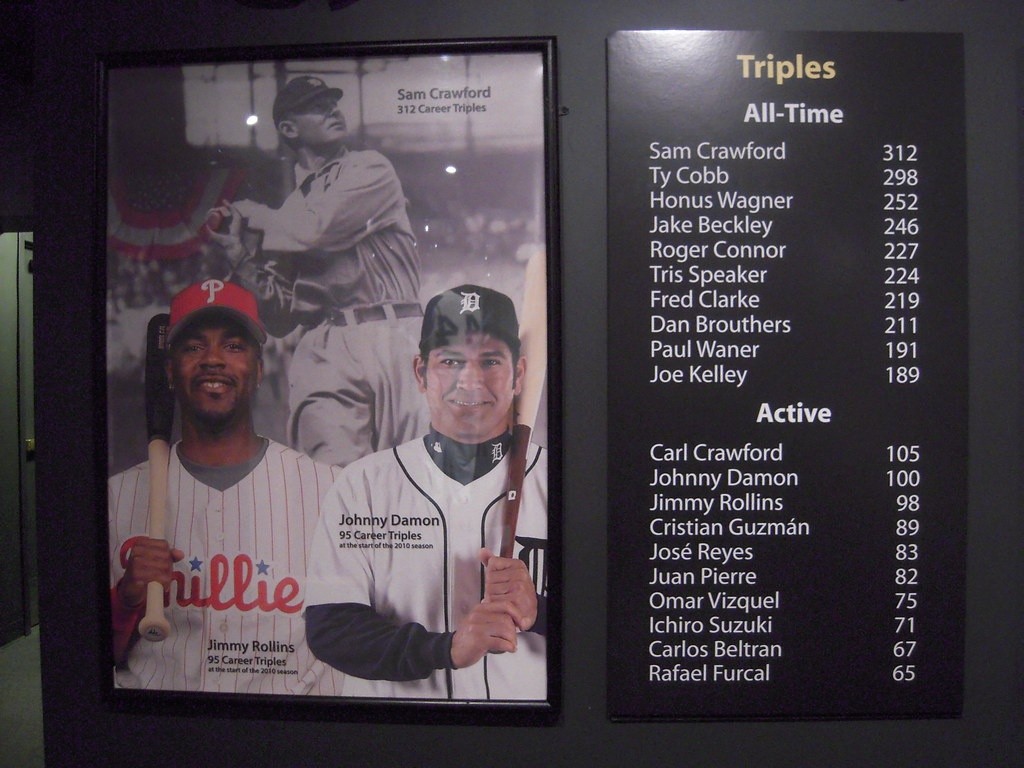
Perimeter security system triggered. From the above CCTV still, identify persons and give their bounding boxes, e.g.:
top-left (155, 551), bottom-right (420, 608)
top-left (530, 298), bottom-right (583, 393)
top-left (204, 77), bottom-right (432, 467)
top-left (304, 285), bottom-right (548, 702)
top-left (105, 278), bottom-right (345, 695)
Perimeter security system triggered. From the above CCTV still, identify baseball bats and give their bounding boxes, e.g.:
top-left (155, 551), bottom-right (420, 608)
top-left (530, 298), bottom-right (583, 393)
top-left (208, 212), bottom-right (232, 231)
top-left (138, 313), bottom-right (171, 642)
top-left (488, 248), bottom-right (547, 654)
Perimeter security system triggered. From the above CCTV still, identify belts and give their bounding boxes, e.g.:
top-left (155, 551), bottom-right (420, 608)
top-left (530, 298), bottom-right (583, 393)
top-left (333, 302), bottom-right (424, 327)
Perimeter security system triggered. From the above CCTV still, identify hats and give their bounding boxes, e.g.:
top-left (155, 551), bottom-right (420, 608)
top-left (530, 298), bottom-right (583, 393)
top-left (166, 279), bottom-right (267, 345)
top-left (419, 284), bottom-right (521, 352)
top-left (272, 76), bottom-right (343, 127)
